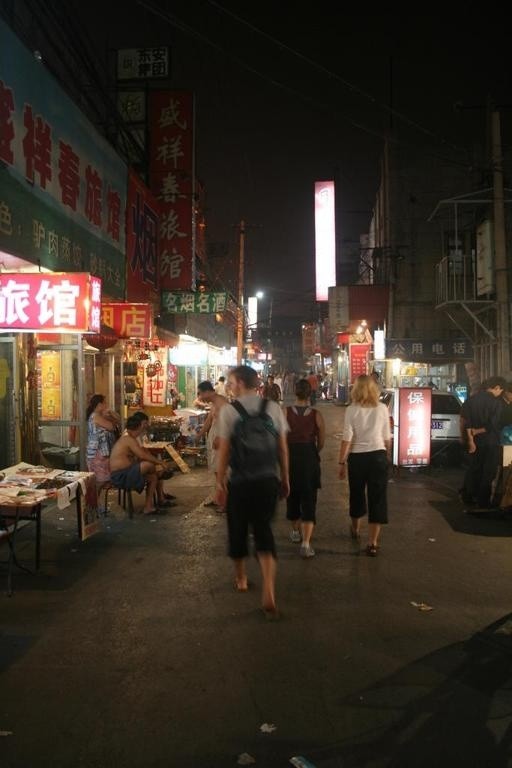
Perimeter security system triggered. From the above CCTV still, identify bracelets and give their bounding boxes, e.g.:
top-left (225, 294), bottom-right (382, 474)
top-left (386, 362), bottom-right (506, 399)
top-left (338, 460), bottom-right (346, 465)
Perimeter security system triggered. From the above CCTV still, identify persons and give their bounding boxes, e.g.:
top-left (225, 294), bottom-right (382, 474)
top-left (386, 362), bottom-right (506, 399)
top-left (456, 375), bottom-right (509, 509)
top-left (280, 376), bottom-right (326, 560)
top-left (84, 392), bottom-right (121, 514)
top-left (191, 379), bottom-right (232, 509)
top-left (493, 380), bottom-right (512, 506)
top-left (370, 370), bottom-right (387, 397)
top-left (336, 374), bottom-right (394, 558)
top-left (109, 415), bottom-right (173, 515)
top-left (191, 365), bottom-right (332, 439)
top-left (210, 364), bottom-right (296, 622)
top-left (121, 410), bottom-right (181, 506)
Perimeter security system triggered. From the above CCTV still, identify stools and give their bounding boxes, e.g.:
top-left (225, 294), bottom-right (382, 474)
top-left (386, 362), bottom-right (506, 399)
top-left (98, 480), bottom-right (157, 519)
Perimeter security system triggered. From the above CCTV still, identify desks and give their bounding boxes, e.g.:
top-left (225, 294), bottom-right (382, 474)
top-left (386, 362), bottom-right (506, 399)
top-left (0, 460), bottom-right (95, 576)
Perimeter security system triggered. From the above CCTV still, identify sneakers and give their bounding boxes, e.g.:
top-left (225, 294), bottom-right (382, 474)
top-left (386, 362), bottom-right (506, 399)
top-left (232, 545), bottom-right (280, 624)
top-left (288, 525), bottom-right (381, 557)
top-left (202, 498), bottom-right (227, 513)
top-left (142, 470), bottom-right (179, 514)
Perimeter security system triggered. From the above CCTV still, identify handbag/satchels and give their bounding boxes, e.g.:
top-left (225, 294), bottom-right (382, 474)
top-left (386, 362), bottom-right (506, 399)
top-left (90, 457), bottom-right (113, 483)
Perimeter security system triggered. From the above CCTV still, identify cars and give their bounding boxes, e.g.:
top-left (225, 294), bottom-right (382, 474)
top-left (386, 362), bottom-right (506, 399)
top-left (380, 386), bottom-right (466, 463)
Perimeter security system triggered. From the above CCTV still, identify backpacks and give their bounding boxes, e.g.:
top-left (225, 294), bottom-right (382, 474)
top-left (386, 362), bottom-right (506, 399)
top-left (226, 394), bottom-right (283, 500)
